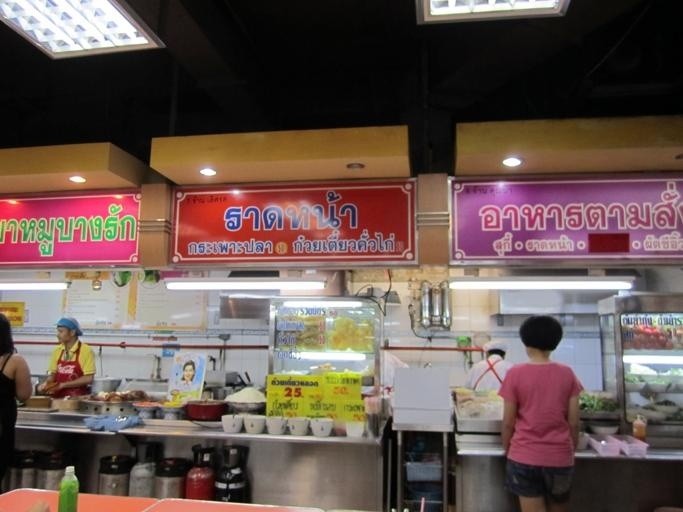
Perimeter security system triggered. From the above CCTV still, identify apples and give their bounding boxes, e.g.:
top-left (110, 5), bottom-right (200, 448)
top-left (622, 324), bottom-right (673, 350)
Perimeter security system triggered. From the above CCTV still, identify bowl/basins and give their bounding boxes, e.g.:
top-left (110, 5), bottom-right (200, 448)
top-left (624, 381), bottom-right (682, 423)
top-left (588, 422), bottom-right (619, 436)
top-left (93, 377), bottom-right (121, 392)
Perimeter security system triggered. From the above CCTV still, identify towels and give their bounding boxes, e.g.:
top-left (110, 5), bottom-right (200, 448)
top-left (84, 414), bottom-right (141, 432)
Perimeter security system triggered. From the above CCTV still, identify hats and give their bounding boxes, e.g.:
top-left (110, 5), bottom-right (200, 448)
top-left (55, 317), bottom-right (83, 336)
top-left (483, 340), bottom-right (509, 353)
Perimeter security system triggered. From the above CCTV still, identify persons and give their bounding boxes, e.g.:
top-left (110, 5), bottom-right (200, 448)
top-left (381, 347), bottom-right (408, 433)
top-left (497, 316), bottom-right (584, 511)
top-left (180, 361), bottom-right (196, 386)
top-left (461, 341), bottom-right (514, 397)
top-left (38, 318), bottom-right (96, 397)
top-left (0, 314), bottom-right (34, 494)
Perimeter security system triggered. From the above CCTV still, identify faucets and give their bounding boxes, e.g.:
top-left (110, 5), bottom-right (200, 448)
top-left (208, 355), bottom-right (216, 369)
top-left (154, 353), bottom-right (162, 377)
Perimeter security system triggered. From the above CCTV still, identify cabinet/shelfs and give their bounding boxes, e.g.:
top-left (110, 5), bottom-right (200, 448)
top-left (598, 289), bottom-right (683, 448)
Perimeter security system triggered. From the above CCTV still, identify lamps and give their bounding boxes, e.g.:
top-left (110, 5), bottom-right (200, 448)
top-left (445, 276), bottom-right (637, 290)
top-left (0, 280), bottom-right (71, 293)
top-left (164, 278), bottom-right (329, 290)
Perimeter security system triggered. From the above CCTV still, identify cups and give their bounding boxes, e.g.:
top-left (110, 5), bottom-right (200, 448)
top-left (221, 413), bottom-right (332, 437)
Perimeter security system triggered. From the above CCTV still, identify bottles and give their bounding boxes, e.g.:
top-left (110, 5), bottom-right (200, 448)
top-left (57, 466), bottom-right (79, 512)
top-left (632, 414), bottom-right (647, 439)
